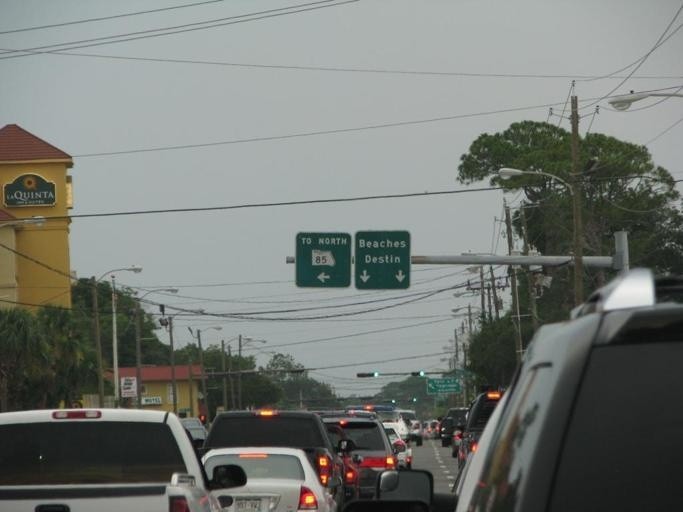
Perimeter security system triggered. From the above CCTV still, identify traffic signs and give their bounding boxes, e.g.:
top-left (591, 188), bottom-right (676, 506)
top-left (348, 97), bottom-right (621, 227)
top-left (354, 230), bottom-right (409, 289)
top-left (295, 232), bottom-right (351, 287)
top-left (425, 377), bottom-right (461, 394)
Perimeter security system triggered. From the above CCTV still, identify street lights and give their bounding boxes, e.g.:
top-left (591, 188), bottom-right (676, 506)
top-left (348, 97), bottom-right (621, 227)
top-left (167, 307), bottom-right (204, 415)
top-left (440, 306), bottom-right (507, 393)
top-left (196, 326), bottom-right (222, 426)
top-left (135, 288), bottom-right (178, 408)
top-left (607, 92), bottom-right (682, 111)
top-left (91, 265), bottom-right (141, 408)
top-left (459, 251), bottom-right (538, 334)
top-left (452, 289), bottom-right (523, 366)
top-left (498, 168), bottom-right (582, 308)
top-left (220, 336), bottom-right (277, 408)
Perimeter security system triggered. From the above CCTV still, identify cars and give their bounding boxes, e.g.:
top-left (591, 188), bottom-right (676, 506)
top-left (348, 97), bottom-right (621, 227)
top-left (312, 404), bottom-right (468, 499)
top-left (174, 415), bottom-right (208, 451)
top-left (197, 446), bottom-right (331, 511)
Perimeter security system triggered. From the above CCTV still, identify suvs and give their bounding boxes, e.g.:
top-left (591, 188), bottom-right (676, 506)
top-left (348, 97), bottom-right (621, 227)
top-left (377, 269), bottom-right (682, 510)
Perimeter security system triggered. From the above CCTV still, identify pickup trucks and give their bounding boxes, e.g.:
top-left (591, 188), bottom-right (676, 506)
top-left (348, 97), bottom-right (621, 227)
top-left (192, 409), bottom-right (355, 510)
top-left (458, 391), bottom-right (506, 471)
top-left (0, 409), bottom-right (223, 511)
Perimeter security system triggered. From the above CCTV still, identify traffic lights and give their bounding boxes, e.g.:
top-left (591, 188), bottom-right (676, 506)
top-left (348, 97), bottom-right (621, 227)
top-left (411, 371), bottom-right (424, 376)
top-left (356, 372), bottom-right (378, 377)
top-left (383, 399), bottom-right (395, 404)
top-left (408, 398), bottom-right (416, 403)
